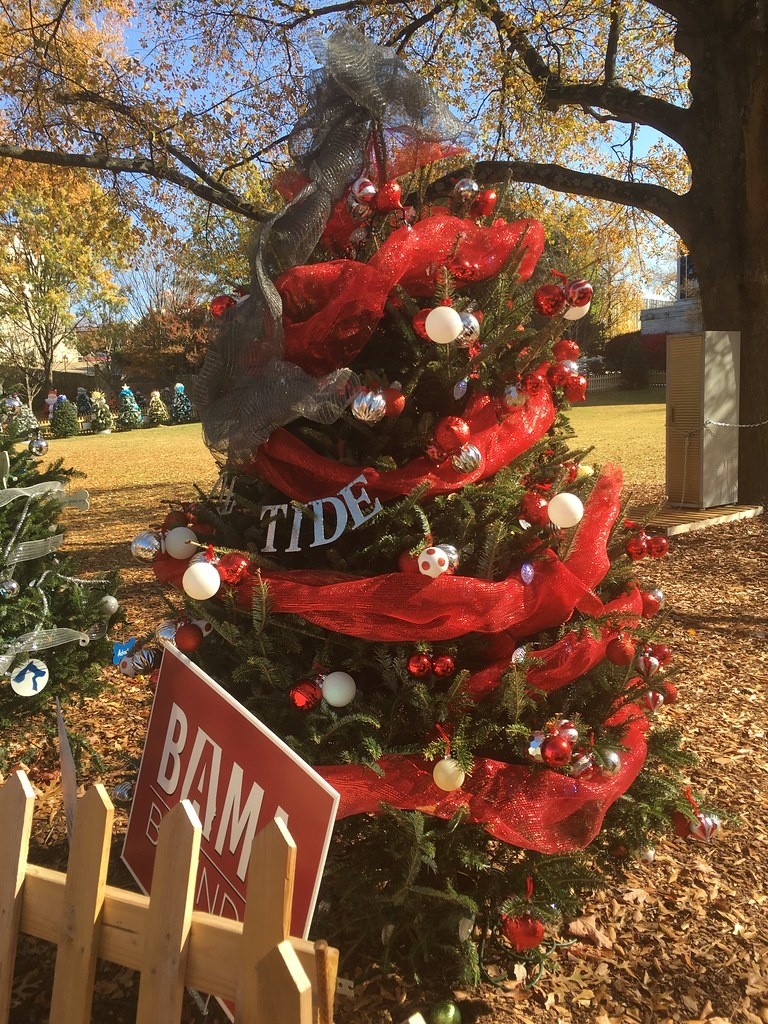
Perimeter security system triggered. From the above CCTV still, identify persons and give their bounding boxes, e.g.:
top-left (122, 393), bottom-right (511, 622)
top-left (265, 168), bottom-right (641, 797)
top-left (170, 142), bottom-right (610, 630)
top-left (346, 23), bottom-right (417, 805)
top-left (44, 391), bottom-right (58, 420)
